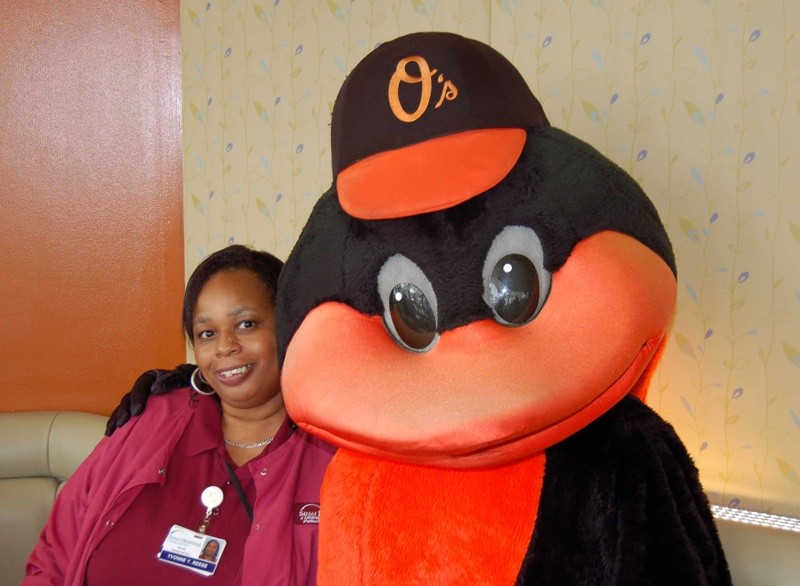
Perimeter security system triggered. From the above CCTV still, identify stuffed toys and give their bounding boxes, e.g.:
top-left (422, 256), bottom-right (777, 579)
top-left (104, 32), bottom-right (733, 586)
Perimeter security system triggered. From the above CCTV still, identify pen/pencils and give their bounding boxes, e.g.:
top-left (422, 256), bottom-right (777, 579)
top-left (226, 463), bottom-right (253, 521)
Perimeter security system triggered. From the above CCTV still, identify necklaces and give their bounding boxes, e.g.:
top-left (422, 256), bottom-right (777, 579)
top-left (224, 436), bottom-right (274, 448)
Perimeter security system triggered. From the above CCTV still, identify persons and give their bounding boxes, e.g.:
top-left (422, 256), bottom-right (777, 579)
top-left (23, 245), bottom-right (336, 586)
top-left (199, 540), bottom-right (219, 562)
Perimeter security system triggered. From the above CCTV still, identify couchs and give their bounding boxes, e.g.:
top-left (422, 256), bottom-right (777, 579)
top-left (0, 409), bottom-right (111, 586)
